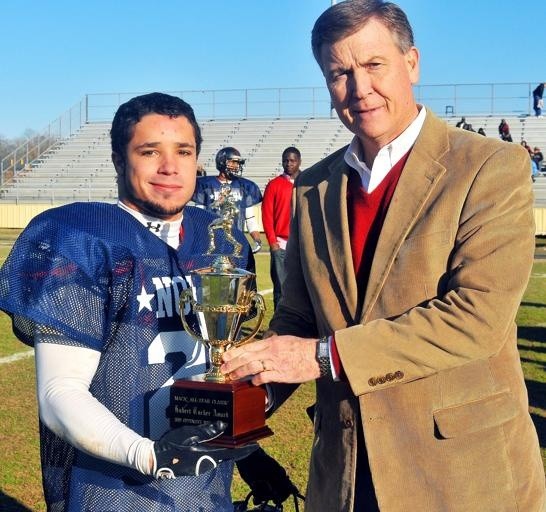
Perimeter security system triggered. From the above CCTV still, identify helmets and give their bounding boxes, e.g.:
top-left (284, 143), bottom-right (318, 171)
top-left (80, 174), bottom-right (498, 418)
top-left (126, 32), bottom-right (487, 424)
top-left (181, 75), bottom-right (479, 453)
top-left (216, 147), bottom-right (245, 181)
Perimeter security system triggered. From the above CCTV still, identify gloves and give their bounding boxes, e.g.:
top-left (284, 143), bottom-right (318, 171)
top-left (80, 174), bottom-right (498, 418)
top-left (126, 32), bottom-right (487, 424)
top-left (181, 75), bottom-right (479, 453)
top-left (251, 239), bottom-right (262, 254)
top-left (149, 421), bottom-right (260, 480)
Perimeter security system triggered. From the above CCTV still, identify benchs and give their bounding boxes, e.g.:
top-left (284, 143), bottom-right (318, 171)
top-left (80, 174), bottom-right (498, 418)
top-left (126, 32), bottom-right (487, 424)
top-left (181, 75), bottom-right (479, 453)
top-left (0, 114), bottom-right (542, 207)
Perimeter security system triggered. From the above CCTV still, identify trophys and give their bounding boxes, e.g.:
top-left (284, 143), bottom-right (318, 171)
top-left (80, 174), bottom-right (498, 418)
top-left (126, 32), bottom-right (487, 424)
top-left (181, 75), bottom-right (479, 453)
top-left (167, 188), bottom-right (276, 450)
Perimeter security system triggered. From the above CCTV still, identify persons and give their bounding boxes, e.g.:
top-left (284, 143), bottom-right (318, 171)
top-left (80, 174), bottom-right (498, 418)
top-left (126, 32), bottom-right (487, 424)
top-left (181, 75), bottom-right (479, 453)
top-left (0, 93), bottom-right (290, 512)
top-left (455, 115), bottom-right (545, 184)
top-left (215, 0), bottom-right (543, 512)
top-left (532, 81), bottom-right (545, 116)
top-left (187, 148), bottom-right (265, 258)
top-left (263, 146), bottom-right (308, 318)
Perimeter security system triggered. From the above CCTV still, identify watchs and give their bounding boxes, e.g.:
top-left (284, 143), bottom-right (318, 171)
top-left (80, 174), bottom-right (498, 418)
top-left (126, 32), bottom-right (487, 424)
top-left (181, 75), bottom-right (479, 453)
top-left (316, 335), bottom-right (334, 379)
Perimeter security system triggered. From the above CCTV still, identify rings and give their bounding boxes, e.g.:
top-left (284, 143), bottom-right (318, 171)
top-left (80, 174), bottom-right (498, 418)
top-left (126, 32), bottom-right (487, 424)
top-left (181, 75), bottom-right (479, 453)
top-left (259, 360), bottom-right (265, 371)
